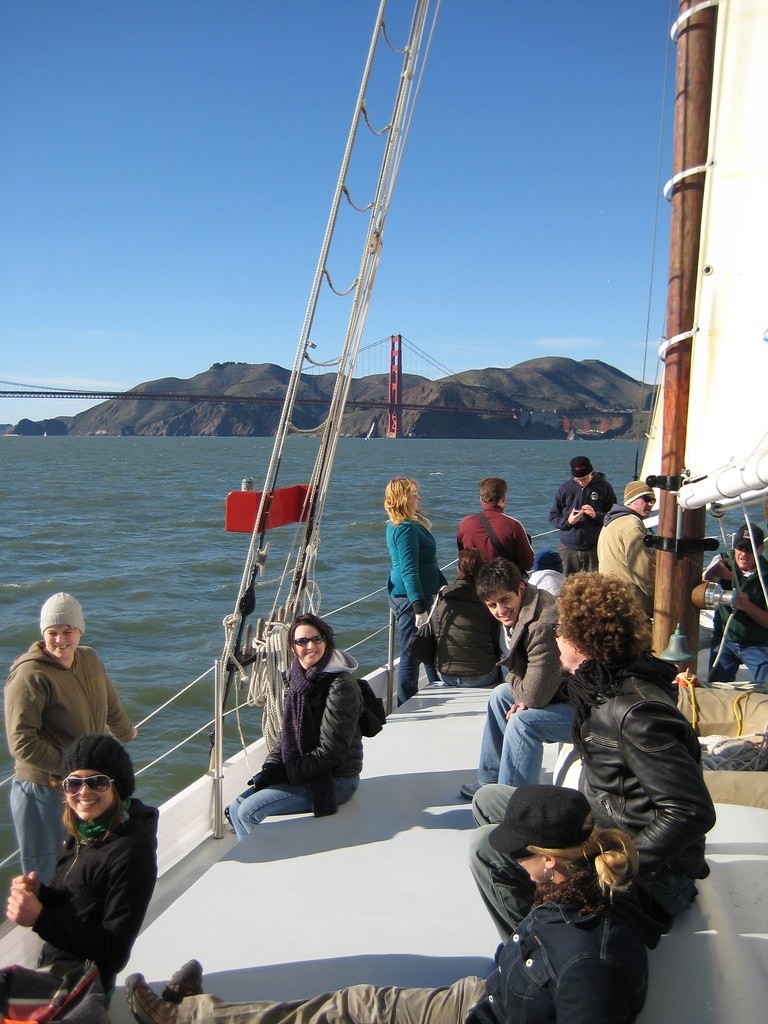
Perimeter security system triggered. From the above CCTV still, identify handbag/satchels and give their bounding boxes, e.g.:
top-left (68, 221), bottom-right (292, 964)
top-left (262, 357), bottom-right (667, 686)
top-left (407, 620), bottom-right (436, 667)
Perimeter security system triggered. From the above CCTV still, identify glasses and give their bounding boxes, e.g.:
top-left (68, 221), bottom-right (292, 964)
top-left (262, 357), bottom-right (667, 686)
top-left (293, 635), bottom-right (326, 646)
top-left (642, 495), bottom-right (656, 503)
top-left (62, 775), bottom-right (115, 795)
top-left (551, 623), bottom-right (563, 639)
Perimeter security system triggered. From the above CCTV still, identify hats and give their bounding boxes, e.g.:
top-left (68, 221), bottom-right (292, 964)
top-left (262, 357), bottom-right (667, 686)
top-left (488, 786), bottom-right (593, 853)
top-left (734, 523), bottom-right (764, 550)
top-left (623, 481), bottom-right (655, 505)
top-left (570, 456), bottom-right (593, 478)
top-left (534, 550), bottom-right (564, 573)
top-left (39, 592), bottom-right (85, 636)
top-left (63, 736), bottom-right (136, 800)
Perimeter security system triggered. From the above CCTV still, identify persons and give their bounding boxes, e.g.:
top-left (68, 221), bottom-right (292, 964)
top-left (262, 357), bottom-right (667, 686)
top-left (435, 455), bottom-right (656, 688)
top-left (702, 523), bottom-right (768, 681)
top-left (384, 476), bottom-right (448, 706)
top-left (0, 734), bottom-right (159, 1018)
top-left (3, 592), bottom-right (136, 885)
top-left (459, 556), bottom-right (716, 963)
top-left (126, 786), bottom-right (648, 1024)
top-left (230, 613), bottom-right (363, 840)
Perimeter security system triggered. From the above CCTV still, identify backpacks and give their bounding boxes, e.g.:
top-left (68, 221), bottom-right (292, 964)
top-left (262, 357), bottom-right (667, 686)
top-left (355, 678), bottom-right (386, 737)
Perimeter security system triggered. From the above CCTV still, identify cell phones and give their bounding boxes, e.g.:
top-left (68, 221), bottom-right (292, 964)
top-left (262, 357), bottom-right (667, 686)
top-left (574, 510), bottom-right (579, 513)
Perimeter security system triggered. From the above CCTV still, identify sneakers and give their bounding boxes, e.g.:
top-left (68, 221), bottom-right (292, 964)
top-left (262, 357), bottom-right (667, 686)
top-left (124, 973), bottom-right (176, 1024)
top-left (161, 959), bottom-right (203, 1005)
top-left (460, 782), bottom-right (483, 800)
